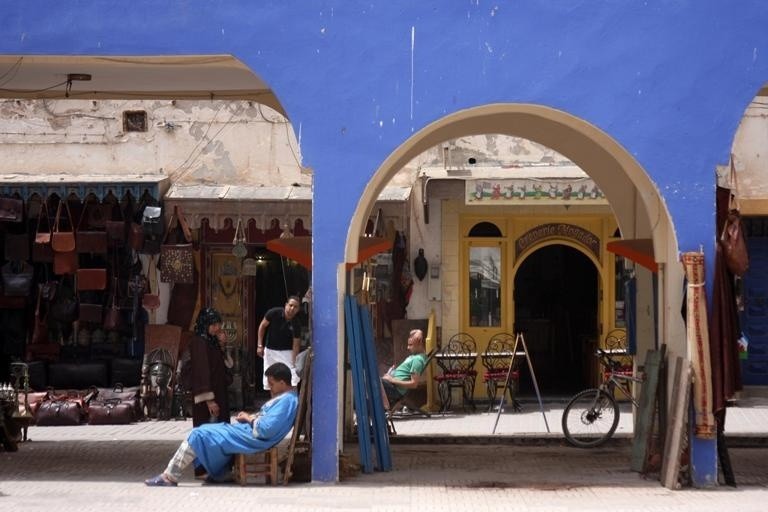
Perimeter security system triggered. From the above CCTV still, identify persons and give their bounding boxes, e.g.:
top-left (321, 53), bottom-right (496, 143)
top-left (352, 328), bottom-right (429, 411)
top-left (188, 307), bottom-right (235, 476)
top-left (141, 361), bottom-right (300, 486)
top-left (256, 295), bottom-right (304, 396)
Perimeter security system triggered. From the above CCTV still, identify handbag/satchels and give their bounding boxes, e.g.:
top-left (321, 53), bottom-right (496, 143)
top-left (88, 398), bottom-right (133, 424)
top-left (128, 222), bottom-right (145, 250)
top-left (5, 234), bottom-right (29, 260)
top-left (104, 306), bottom-right (120, 330)
top-left (31, 314), bottom-right (50, 345)
top-left (35, 399), bottom-right (82, 425)
top-left (97, 383), bottom-right (140, 418)
top-left (79, 303), bottom-right (102, 323)
top-left (141, 293), bottom-right (160, 309)
top-left (160, 244), bottom-right (194, 284)
top-left (3, 274), bottom-right (31, 296)
top-left (77, 269), bottom-right (107, 291)
top-left (107, 221), bottom-right (125, 241)
top-left (52, 232), bottom-right (76, 252)
top-left (53, 251), bottom-right (80, 275)
top-left (33, 232), bottom-right (56, 263)
top-left (0, 198), bottom-right (23, 222)
top-left (76, 231), bottom-right (107, 254)
top-left (39, 281), bottom-right (77, 316)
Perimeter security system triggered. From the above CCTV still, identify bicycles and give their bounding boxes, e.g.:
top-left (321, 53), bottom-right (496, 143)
top-left (562, 347), bottom-right (643, 448)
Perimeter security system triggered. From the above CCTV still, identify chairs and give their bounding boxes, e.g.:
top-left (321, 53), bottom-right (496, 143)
top-left (352, 330), bottom-right (532, 421)
top-left (602, 330), bottom-right (636, 405)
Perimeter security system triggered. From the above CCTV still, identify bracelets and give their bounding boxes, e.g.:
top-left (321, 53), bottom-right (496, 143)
top-left (258, 344), bottom-right (263, 348)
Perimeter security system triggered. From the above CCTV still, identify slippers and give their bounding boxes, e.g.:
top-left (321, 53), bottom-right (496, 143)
top-left (144, 474), bottom-right (177, 487)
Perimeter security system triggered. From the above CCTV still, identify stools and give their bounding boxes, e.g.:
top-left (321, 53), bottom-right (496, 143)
top-left (234, 447), bottom-right (278, 487)
